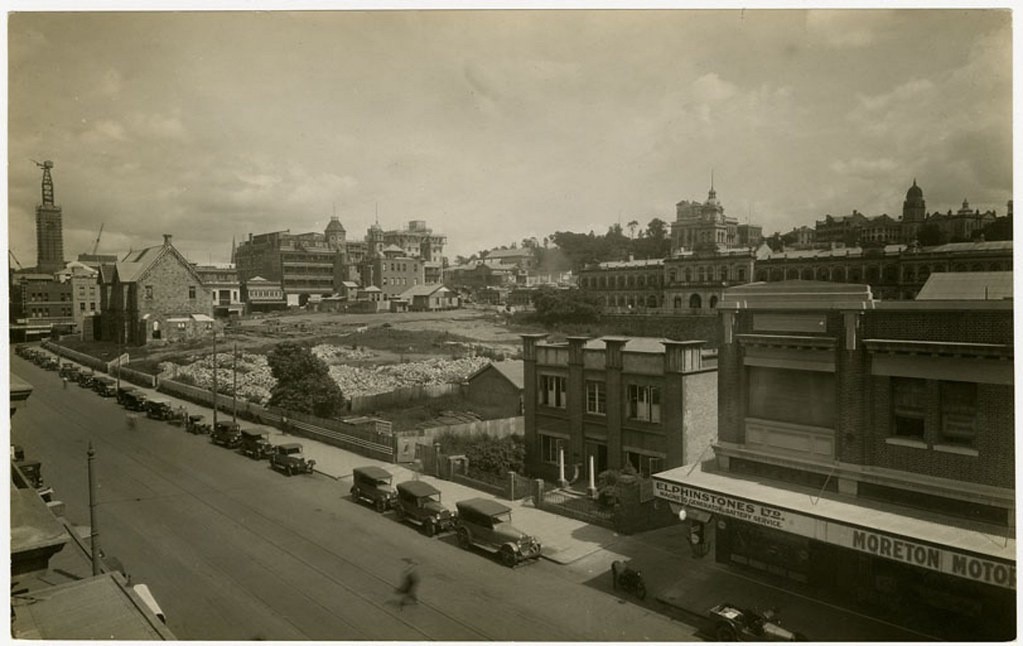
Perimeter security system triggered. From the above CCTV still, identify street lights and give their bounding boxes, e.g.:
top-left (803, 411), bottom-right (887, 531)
top-left (508, 472), bottom-right (515, 502)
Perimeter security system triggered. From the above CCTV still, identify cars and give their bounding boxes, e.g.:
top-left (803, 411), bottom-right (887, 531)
top-left (270, 443), bottom-right (308, 476)
top-left (211, 422), bottom-right (243, 450)
top-left (242, 428), bottom-right (276, 460)
top-left (350, 466), bottom-right (396, 513)
top-left (396, 480), bottom-right (457, 537)
top-left (455, 498), bottom-right (542, 567)
top-left (15, 342), bottom-right (249, 436)
top-left (696, 603), bottom-right (795, 642)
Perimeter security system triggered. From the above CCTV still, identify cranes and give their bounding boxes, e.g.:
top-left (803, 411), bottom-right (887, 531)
top-left (94, 222), bottom-right (104, 256)
top-left (28, 158), bottom-right (54, 205)
